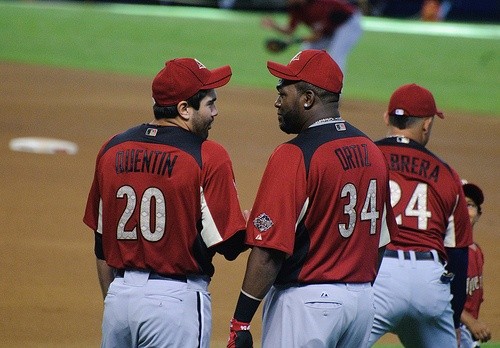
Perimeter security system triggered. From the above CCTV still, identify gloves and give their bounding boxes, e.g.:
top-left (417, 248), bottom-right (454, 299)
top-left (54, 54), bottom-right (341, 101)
top-left (227, 317), bottom-right (254, 348)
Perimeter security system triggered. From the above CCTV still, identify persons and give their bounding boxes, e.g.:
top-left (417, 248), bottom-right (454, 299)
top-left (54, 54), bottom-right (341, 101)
top-left (226, 48), bottom-right (398, 348)
top-left (83, 58), bottom-right (253, 348)
top-left (457, 179), bottom-right (492, 348)
top-left (357, 84), bottom-right (470, 348)
top-left (213, 0), bottom-right (500, 88)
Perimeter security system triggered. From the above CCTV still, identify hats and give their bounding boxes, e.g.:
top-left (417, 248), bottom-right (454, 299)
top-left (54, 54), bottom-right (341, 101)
top-left (388, 83), bottom-right (444, 119)
top-left (266, 50), bottom-right (343, 94)
top-left (461, 179), bottom-right (484, 205)
top-left (152, 58), bottom-right (232, 107)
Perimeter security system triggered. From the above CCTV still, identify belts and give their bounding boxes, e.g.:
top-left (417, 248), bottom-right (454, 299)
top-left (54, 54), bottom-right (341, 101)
top-left (383, 250), bottom-right (443, 262)
top-left (113, 268), bottom-right (187, 283)
